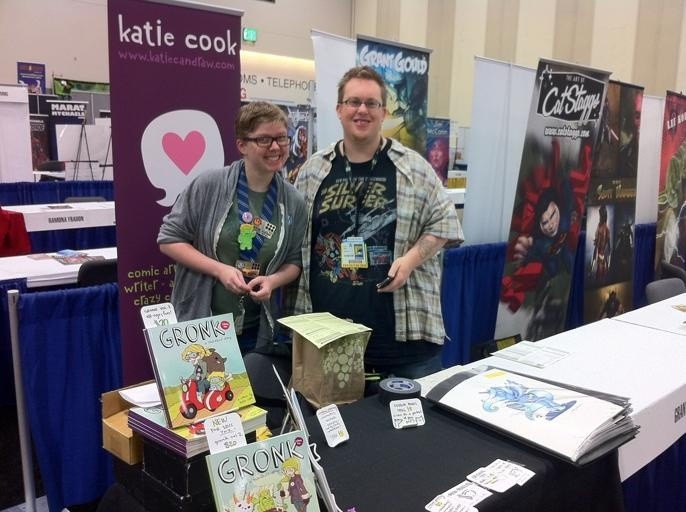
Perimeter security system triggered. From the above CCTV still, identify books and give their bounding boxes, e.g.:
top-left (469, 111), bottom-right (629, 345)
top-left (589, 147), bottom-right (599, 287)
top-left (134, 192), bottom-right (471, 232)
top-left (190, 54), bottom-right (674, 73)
top-left (125, 308), bottom-right (321, 512)
top-left (422, 363), bottom-right (643, 467)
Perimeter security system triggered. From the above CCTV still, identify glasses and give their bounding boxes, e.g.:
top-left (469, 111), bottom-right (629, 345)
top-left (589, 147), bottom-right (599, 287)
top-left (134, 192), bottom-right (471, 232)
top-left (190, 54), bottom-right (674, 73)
top-left (242, 135), bottom-right (292, 148)
top-left (340, 96), bottom-right (384, 112)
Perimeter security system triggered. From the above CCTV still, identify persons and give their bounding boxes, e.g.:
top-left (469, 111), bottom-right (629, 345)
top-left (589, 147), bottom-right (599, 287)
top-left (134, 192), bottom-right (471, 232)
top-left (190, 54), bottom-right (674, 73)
top-left (155, 99), bottom-right (308, 362)
top-left (591, 203), bottom-right (612, 274)
top-left (512, 185), bottom-right (581, 279)
top-left (424, 135), bottom-right (451, 186)
top-left (285, 64), bottom-right (466, 389)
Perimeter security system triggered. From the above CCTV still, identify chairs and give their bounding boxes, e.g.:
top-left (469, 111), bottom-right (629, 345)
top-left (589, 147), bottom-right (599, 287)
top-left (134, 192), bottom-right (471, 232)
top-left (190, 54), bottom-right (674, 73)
top-left (645, 278), bottom-right (685, 303)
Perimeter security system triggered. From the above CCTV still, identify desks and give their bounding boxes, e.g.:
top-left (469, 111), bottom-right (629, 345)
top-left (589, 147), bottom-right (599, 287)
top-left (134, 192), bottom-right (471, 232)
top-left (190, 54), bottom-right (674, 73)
top-left (115, 290), bottom-right (686, 512)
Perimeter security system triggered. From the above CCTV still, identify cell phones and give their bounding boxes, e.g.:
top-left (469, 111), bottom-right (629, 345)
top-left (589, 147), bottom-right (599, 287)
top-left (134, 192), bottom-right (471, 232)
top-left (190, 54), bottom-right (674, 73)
top-left (376, 277), bottom-right (392, 288)
